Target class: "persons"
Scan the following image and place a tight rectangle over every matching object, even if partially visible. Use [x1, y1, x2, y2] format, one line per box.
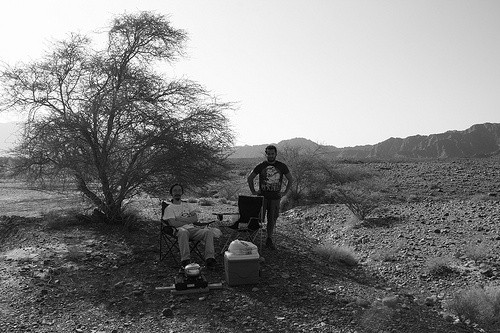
[162, 184, 218, 270]
[249, 145, 293, 247]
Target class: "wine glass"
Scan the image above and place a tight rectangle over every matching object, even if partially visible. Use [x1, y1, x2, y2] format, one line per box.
[217, 214, 223, 226]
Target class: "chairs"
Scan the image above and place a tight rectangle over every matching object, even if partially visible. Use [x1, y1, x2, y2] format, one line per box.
[159, 201, 216, 267]
[218, 195, 264, 256]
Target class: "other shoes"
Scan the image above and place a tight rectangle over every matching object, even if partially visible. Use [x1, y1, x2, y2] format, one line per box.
[267, 238, 278, 249]
[207, 259, 216, 267]
[181, 260, 190, 269]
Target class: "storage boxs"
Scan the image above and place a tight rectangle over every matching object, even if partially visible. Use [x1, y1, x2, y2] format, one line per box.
[222, 250, 261, 279]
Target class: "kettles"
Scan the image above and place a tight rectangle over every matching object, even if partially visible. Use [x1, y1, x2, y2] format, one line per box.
[181, 259, 206, 277]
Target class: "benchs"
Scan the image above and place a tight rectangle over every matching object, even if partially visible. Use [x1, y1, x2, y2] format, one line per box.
[212, 212, 240, 221]
